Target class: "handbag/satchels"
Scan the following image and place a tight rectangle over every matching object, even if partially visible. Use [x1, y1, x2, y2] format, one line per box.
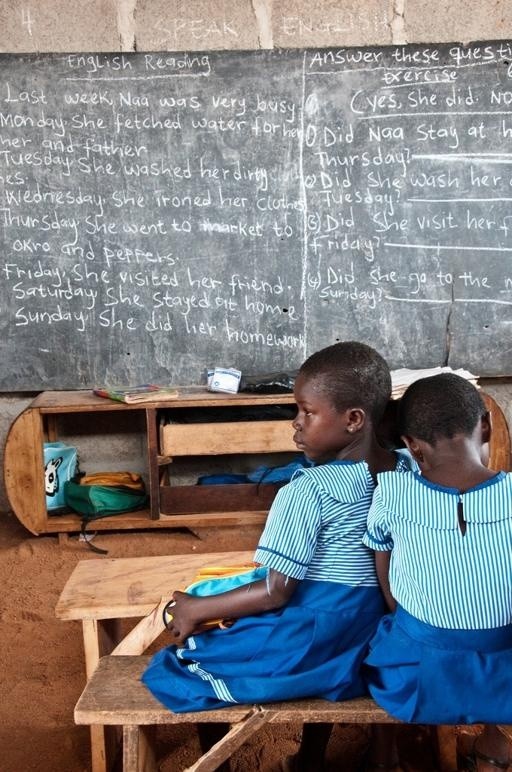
[246, 462, 308, 484]
[43, 442, 79, 511]
[196, 474, 251, 484]
[185, 560, 271, 629]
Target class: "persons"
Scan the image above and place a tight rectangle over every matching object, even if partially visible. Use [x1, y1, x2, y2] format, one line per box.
[142, 342, 412, 713]
[362, 373, 512, 724]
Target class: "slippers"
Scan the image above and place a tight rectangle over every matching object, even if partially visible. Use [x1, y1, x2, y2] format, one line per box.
[363, 756, 402, 772]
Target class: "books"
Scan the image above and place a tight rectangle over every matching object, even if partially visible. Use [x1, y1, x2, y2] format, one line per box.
[84, 380, 178, 404]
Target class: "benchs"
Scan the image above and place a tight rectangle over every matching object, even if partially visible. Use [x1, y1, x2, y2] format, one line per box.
[53, 551, 464, 769]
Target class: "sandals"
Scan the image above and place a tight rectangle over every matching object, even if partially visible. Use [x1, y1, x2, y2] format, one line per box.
[457, 731, 511, 771]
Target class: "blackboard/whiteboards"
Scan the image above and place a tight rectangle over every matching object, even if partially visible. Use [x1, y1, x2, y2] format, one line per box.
[0, 39, 512, 393]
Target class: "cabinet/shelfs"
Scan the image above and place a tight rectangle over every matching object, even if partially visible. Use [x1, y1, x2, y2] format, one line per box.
[5, 380, 511, 545]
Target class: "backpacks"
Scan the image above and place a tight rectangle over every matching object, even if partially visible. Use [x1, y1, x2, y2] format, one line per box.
[64, 471, 149, 519]
